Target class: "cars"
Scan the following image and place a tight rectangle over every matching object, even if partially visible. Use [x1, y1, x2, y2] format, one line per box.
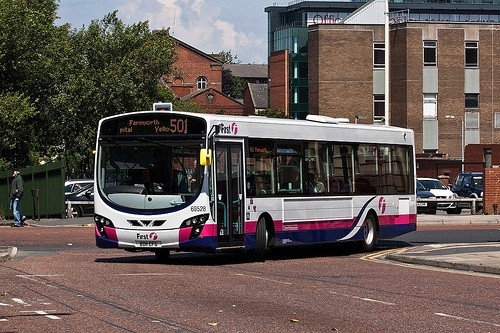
[416, 177, 461, 214]
[65, 178, 94, 195]
[416, 180, 437, 214]
[65, 182, 94, 219]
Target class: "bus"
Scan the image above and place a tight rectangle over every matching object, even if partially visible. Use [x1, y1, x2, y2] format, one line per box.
[93, 102, 417, 263]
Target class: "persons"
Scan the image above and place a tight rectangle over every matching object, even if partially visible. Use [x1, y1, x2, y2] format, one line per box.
[187, 160, 197, 192]
[9, 168, 26, 228]
[308, 173, 325, 193]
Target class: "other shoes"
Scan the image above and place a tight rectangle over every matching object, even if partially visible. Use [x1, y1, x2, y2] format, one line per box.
[11, 225, 21, 227]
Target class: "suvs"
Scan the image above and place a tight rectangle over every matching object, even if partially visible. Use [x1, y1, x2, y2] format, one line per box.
[448, 171, 484, 210]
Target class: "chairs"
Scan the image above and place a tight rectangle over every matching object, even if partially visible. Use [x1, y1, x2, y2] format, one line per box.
[306, 177, 352, 194]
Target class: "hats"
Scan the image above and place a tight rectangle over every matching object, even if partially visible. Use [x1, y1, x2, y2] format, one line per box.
[12, 168, 19, 171]
